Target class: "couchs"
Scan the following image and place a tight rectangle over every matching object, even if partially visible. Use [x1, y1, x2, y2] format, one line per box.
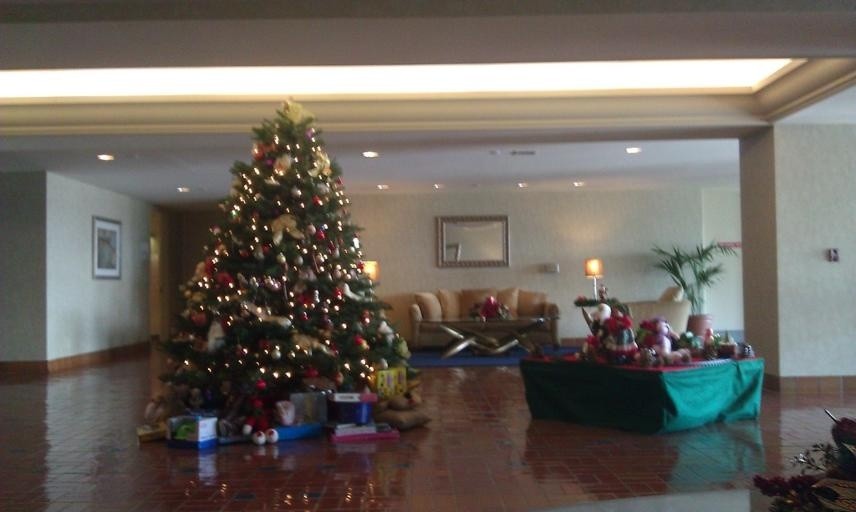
[410, 287, 562, 351]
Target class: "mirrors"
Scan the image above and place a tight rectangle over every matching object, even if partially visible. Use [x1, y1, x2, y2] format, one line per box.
[437, 215, 511, 268]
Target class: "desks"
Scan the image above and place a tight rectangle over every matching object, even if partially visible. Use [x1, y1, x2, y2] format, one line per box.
[519, 356, 766, 435]
[411, 317, 557, 362]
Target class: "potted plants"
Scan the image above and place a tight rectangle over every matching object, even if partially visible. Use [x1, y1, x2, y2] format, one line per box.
[649, 241, 744, 339]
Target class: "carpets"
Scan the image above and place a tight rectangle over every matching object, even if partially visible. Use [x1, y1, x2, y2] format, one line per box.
[405, 345, 580, 370]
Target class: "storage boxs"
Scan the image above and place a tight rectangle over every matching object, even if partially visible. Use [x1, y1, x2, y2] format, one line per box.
[164, 414, 218, 451]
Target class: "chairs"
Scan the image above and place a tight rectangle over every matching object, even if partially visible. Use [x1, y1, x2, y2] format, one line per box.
[624, 285, 692, 334]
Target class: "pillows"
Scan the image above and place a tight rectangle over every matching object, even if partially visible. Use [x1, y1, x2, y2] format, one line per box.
[416, 288, 547, 318]
[656, 286, 684, 302]
[376, 409, 431, 430]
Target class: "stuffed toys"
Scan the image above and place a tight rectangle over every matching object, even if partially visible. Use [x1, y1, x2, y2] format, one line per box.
[580, 304, 756, 369]
[241, 394, 279, 445]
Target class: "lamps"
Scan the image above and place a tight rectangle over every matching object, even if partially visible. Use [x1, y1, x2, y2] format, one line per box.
[363, 259, 376, 282]
[584, 257, 604, 298]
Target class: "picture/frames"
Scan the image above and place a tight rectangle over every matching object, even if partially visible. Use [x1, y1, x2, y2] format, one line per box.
[90, 215, 120, 280]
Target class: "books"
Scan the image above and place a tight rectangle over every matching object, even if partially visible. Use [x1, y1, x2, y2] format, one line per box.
[324, 422, 376, 437]
